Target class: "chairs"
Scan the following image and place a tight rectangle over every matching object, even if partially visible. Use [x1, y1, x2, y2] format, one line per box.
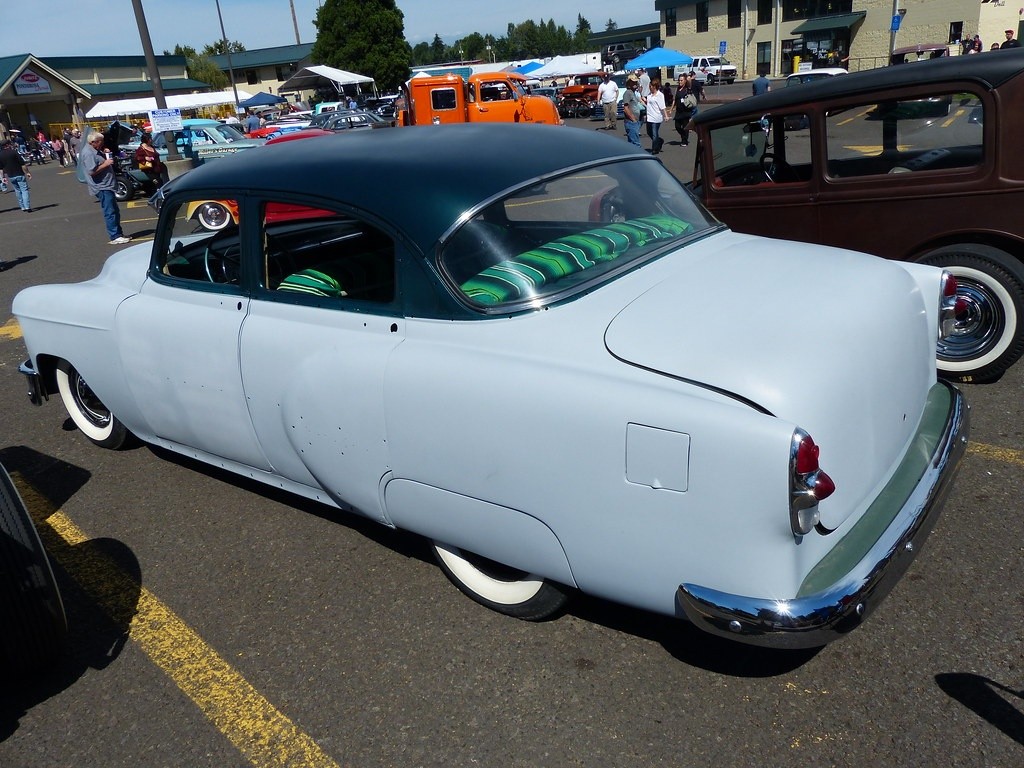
[276, 255, 377, 310]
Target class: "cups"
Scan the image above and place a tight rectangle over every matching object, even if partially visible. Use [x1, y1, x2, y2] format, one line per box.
[105, 152, 113, 159]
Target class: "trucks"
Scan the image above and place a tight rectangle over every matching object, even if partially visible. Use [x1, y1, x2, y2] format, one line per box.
[397, 71, 564, 126]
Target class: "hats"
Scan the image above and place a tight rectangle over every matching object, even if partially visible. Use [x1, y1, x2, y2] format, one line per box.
[625, 74, 639, 83]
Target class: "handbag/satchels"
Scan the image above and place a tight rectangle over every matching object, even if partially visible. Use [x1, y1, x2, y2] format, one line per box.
[139, 161, 152, 169]
[680, 94, 697, 109]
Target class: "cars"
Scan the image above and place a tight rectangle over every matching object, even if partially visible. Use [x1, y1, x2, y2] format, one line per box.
[587, 44, 1024, 385]
[184, 128, 340, 232]
[488, 72, 644, 120]
[9, 119, 971, 652]
[72, 94, 406, 201]
[783, 67, 849, 118]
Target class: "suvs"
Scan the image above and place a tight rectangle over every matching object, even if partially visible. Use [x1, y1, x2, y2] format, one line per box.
[600, 43, 645, 66]
[672, 54, 738, 86]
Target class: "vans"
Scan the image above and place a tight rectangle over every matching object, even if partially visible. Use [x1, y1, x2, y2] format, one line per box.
[886, 43, 954, 117]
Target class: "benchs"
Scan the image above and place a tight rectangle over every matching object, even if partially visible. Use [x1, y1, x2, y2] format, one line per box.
[458, 214, 694, 311]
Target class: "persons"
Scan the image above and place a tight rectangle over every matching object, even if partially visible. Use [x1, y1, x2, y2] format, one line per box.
[11, 127, 82, 168]
[549, 76, 557, 87]
[752, 68, 771, 96]
[827, 50, 850, 67]
[597, 73, 619, 130]
[961, 34, 983, 55]
[226, 111, 240, 124]
[622, 67, 707, 154]
[0, 139, 33, 213]
[82, 132, 133, 244]
[990, 30, 1020, 51]
[134, 133, 163, 197]
[137, 120, 151, 127]
[246, 111, 266, 133]
[566, 74, 576, 86]
[308, 94, 318, 107]
[347, 97, 358, 109]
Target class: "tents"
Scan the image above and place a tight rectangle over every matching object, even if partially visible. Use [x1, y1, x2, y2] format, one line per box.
[398, 71, 430, 94]
[624, 47, 693, 88]
[238, 92, 288, 121]
[277, 66, 379, 102]
[498, 55, 603, 87]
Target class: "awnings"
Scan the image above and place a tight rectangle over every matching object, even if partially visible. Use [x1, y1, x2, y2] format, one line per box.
[790, 13, 866, 35]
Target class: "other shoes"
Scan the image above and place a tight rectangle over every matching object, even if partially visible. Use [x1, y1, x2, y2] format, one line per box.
[26, 209, 32, 212]
[110, 235, 132, 244]
[681, 142, 687, 146]
